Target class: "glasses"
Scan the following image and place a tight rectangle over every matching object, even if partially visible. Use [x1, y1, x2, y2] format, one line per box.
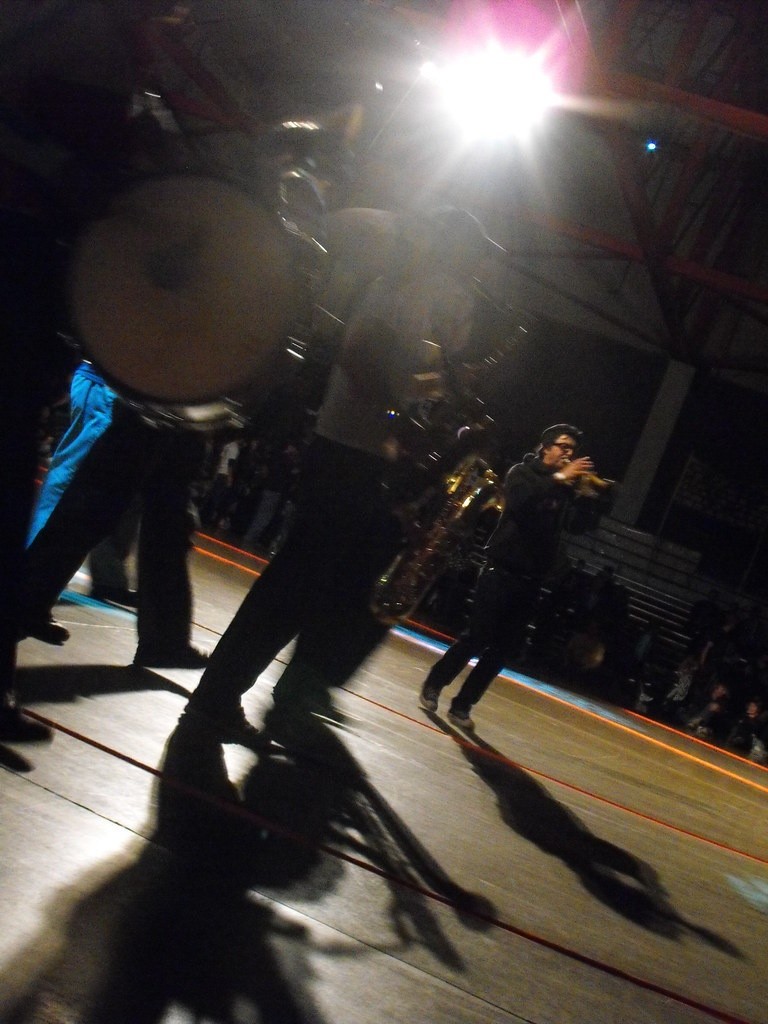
[549, 442, 578, 453]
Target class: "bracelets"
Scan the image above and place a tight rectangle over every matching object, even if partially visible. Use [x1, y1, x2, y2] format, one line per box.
[552, 472, 566, 481]
[421, 338, 441, 368]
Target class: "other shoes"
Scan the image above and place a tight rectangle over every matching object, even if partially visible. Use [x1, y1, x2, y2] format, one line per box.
[90, 586, 138, 607]
[134, 644, 212, 669]
[31, 623, 70, 642]
[272, 672, 345, 722]
[0, 709, 51, 742]
[183, 693, 261, 738]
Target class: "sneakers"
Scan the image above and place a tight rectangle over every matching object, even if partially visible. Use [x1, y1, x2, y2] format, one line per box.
[422, 682, 439, 710]
[447, 707, 474, 729]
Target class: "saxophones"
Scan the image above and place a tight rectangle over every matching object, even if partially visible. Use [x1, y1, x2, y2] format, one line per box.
[366, 450, 507, 623]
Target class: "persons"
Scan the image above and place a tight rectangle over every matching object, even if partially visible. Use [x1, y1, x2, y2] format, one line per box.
[0, 0, 503, 754]
[421, 421, 597, 732]
[506, 555, 768, 767]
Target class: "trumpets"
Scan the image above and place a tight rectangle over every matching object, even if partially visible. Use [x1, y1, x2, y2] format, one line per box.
[558, 457, 616, 496]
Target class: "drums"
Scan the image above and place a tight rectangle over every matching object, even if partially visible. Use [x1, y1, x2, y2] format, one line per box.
[69, 175, 297, 399]
